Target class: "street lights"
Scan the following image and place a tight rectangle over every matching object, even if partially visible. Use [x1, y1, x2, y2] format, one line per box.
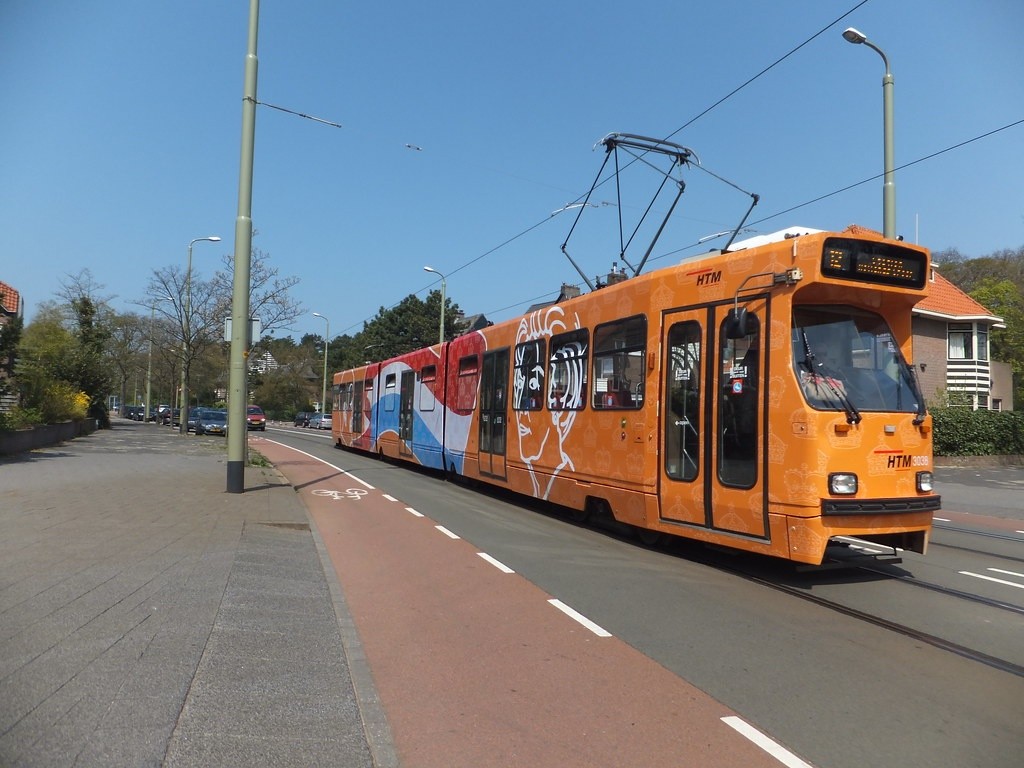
[312, 313, 330, 414]
[146, 298, 175, 422]
[424, 266, 449, 346]
[842, 27, 897, 241]
[225, 97, 343, 493]
[178, 237, 222, 434]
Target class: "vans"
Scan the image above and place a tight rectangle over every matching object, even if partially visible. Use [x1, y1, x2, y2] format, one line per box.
[246, 405, 266, 432]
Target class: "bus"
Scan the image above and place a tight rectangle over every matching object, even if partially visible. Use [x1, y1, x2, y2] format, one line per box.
[332, 132, 943, 566]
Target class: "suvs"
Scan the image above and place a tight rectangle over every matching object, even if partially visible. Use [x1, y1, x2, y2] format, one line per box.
[294, 412, 316, 429]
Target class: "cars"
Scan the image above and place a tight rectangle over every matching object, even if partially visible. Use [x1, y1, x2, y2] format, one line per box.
[123, 405, 180, 426]
[186, 407, 209, 432]
[195, 411, 227, 436]
[308, 414, 333, 431]
